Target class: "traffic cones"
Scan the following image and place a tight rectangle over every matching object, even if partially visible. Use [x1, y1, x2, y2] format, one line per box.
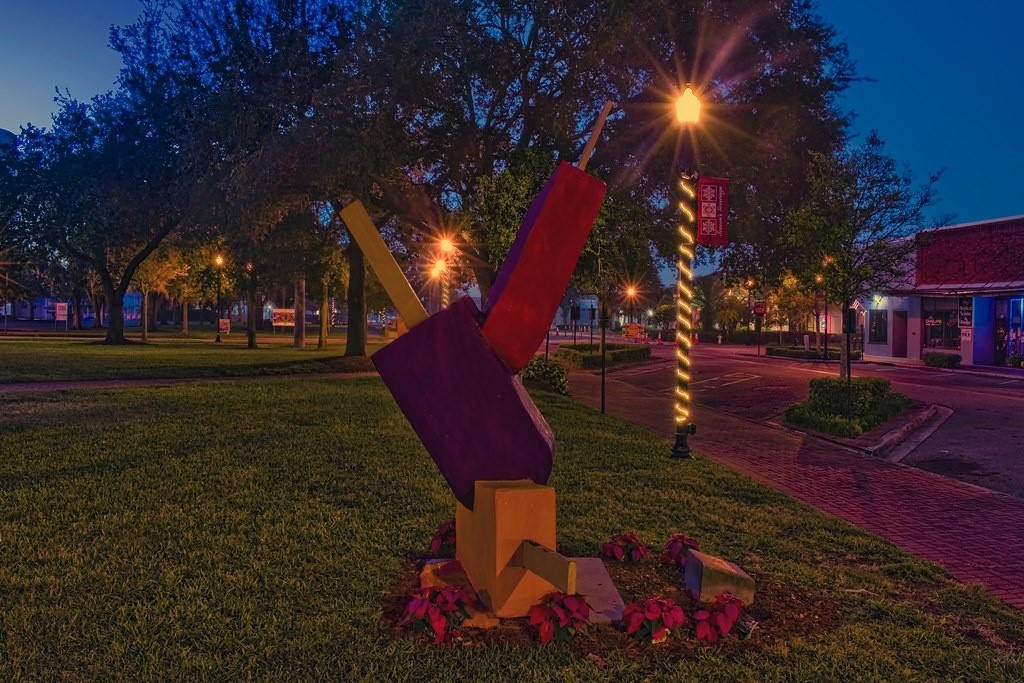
[555, 331, 559, 336]
[656, 334, 664, 345]
[673, 335, 681, 347]
[693, 334, 699, 345]
[642, 333, 649, 344]
[635, 332, 641, 343]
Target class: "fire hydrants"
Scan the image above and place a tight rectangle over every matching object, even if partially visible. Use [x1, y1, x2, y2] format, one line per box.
[716, 335, 722, 344]
[793, 338, 799, 349]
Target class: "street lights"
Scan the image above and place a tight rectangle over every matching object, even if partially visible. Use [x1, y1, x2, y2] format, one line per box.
[214, 256, 223, 343]
[628, 287, 634, 323]
[746, 280, 754, 331]
[668, 86, 704, 462]
[431, 239, 455, 312]
